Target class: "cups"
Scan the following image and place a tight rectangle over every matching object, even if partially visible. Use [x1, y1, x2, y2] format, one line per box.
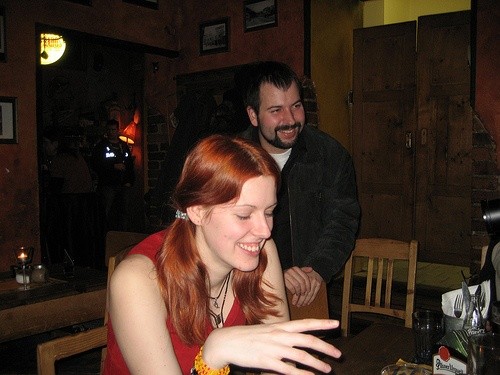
[412, 311, 445, 366]
[379, 363, 426, 375]
[466, 331, 500, 375]
[441, 314, 465, 336]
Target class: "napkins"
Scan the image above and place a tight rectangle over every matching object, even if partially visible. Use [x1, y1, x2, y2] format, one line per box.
[440, 279, 491, 320]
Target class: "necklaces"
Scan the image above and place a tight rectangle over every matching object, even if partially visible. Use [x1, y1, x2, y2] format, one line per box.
[207, 271, 231, 328]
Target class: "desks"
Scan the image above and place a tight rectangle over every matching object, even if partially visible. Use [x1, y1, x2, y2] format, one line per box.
[311, 322, 417, 375]
[0, 261, 107, 343]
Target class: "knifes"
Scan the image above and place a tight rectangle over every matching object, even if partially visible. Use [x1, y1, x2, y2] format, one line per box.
[464, 284, 481, 326]
[461, 280, 472, 313]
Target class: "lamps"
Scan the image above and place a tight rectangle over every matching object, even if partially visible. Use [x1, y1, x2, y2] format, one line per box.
[119, 121, 136, 146]
[39, 32, 67, 65]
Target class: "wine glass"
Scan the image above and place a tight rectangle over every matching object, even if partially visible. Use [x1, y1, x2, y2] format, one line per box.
[13, 234, 37, 290]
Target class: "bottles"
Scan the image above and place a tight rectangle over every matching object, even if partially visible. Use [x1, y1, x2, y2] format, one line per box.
[30, 265, 49, 284]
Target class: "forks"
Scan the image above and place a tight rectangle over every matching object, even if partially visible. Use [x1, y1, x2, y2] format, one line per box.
[454, 294, 463, 318]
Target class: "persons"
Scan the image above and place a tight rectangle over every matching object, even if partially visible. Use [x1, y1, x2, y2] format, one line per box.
[98, 119, 136, 232]
[104, 136, 341, 375]
[239, 61, 361, 308]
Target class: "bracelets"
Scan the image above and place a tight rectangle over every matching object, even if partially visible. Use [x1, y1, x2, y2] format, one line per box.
[190, 345, 230, 375]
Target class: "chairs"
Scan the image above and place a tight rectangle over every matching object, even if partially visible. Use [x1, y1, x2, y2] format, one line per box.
[340, 238, 418, 337]
[36, 231, 150, 375]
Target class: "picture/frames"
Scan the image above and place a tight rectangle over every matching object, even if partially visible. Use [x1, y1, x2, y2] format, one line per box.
[0, 96, 19, 144]
[242, 0, 278, 33]
[0, 6, 8, 63]
[199, 16, 228, 55]
